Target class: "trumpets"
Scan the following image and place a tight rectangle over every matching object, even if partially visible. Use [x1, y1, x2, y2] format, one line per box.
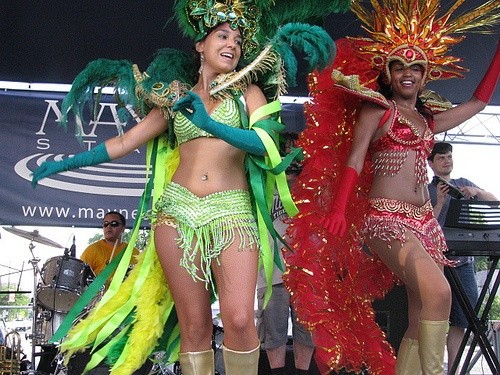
[0, 330, 21, 374]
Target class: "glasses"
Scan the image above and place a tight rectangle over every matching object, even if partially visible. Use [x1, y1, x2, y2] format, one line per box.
[101, 220, 123, 228]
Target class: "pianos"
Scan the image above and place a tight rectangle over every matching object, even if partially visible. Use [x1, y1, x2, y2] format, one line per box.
[436, 225, 500, 375]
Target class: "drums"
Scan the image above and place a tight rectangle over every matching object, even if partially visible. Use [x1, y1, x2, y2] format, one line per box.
[33, 304, 87, 348]
[62, 311, 163, 375]
[37, 255, 99, 313]
[124, 265, 133, 283]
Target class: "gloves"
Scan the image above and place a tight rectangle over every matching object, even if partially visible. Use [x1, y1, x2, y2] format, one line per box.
[31, 140, 112, 187]
[172, 90, 269, 156]
[473, 43, 500, 103]
[322, 166, 359, 236]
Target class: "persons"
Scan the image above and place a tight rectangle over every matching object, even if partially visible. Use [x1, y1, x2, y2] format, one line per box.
[427, 142, 500, 375]
[80, 211, 140, 286]
[257, 131, 317, 375]
[30, 0, 337, 375]
[281, 0, 500, 375]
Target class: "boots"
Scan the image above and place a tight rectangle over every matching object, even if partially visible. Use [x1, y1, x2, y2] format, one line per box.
[222, 338, 262, 375]
[396, 336, 424, 375]
[418, 319, 448, 375]
[178, 349, 214, 375]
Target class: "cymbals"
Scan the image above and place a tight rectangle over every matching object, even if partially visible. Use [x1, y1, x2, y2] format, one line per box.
[1, 225, 66, 250]
